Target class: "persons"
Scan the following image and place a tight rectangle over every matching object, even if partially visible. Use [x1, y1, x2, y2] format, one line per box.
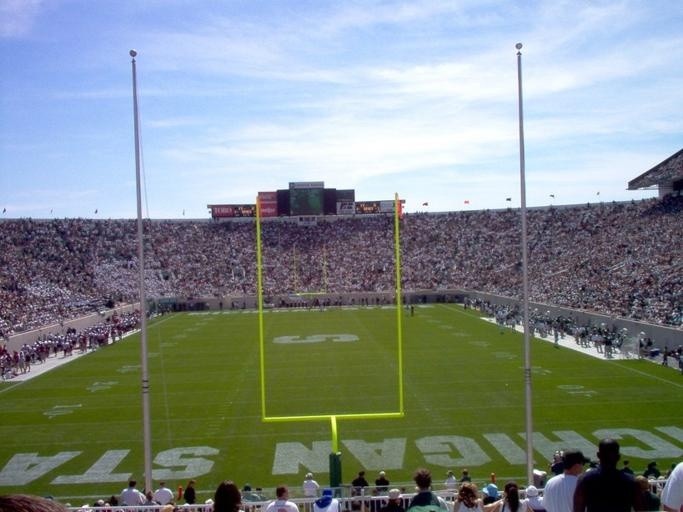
[470, 192, 683, 372]
[213, 439, 683, 512]
[0, 216, 174, 380]
[238, 206, 243, 216]
[174, 214, 470, 316]
[1, 480, 214, 512]
[251, 206, 255, 215]
[372, 203, 380, 214]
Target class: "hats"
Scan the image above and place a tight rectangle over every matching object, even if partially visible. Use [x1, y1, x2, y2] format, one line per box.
[525, 485, 538, 497]
[562, 452, 590, 469]
[388, 489, 402, 499]
[482, 483, 500, 498]
[305, 472, 313, 478]
[322, 488, 333, 495]
